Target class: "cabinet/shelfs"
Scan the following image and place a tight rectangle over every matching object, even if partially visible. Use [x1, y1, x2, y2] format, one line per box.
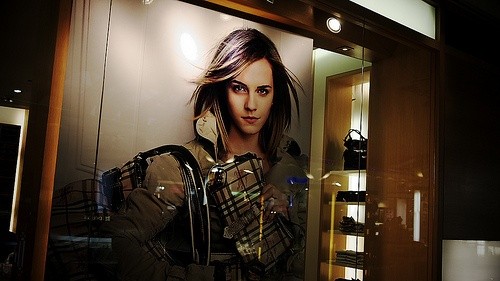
[328, 170, 366, 281]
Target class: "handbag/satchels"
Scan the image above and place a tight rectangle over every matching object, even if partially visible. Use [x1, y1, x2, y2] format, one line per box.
[204, 150, 301, 279]
[342, 128, 367, 152]
[47, 144, 209, 281]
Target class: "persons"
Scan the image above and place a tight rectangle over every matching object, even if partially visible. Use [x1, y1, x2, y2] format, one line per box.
[102, 26, 310, 281]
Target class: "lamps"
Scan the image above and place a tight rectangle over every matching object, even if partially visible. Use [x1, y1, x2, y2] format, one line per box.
[326, 17, 345, 34]
